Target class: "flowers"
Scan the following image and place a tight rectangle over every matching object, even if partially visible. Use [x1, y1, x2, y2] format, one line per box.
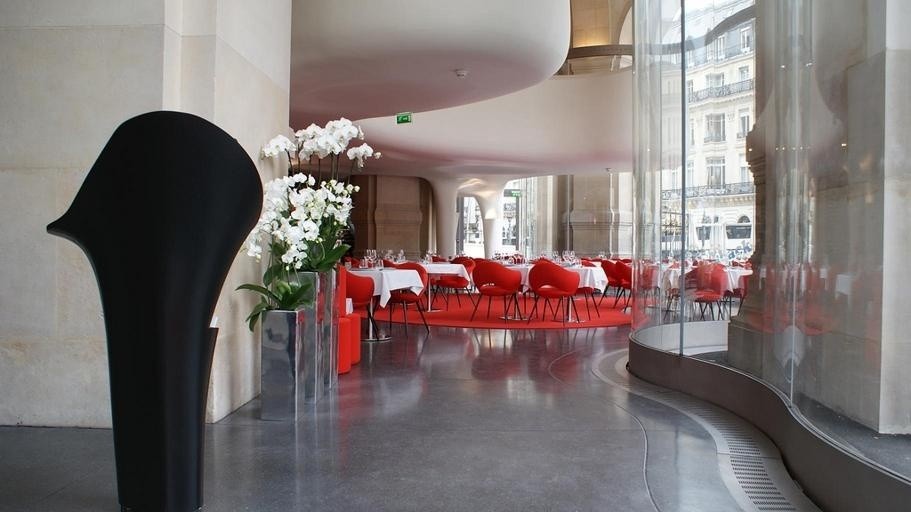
[234, 117, 382, 332]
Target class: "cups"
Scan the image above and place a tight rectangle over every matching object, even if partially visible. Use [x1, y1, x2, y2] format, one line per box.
[344, 261, 353, 271]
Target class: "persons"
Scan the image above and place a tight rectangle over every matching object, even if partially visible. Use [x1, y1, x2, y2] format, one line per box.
[342, 217, 356, 267]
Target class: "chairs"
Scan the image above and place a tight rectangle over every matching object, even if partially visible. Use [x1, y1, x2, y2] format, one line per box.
[297, 253, 758, 374]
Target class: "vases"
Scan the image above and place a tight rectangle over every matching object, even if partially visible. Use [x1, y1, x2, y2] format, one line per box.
[258, 264, 341, 422]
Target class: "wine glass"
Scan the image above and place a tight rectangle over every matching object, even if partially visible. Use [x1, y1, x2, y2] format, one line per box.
[359, 248, 433, 271]
[491, 249, 583, 268]
